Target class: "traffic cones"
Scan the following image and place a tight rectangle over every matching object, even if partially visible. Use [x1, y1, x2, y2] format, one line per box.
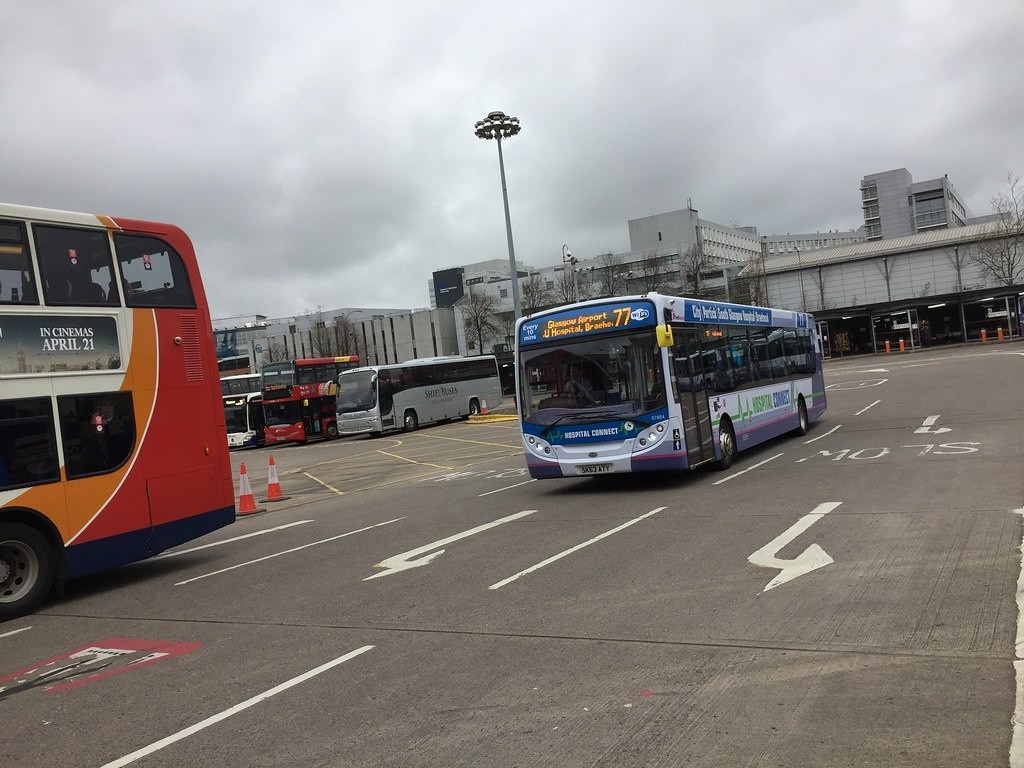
[234, 462, 267, 517]
[259, 455, 291, 503]
[481, 400, 488, 415]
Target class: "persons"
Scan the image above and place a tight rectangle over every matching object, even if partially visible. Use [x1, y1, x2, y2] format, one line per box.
[563, 366, 595, 407]
[784, 360, 797, 375]
[944, 323, 949, 343]
[920, 319, 930, 347]
[21, 247, 131, 304]
[41, 427, 75, 479]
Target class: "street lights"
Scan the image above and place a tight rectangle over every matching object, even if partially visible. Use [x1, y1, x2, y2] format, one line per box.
[346, 310, 362, 327]
[475, 112, 522, 414]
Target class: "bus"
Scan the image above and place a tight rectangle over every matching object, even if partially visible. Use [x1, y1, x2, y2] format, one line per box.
[219, 371, 293, 447]
[335, 352, 502, 437]
[0, 201, 236, 622]
[260, 356, 359, 445]
[515, 293, 828, 479]
